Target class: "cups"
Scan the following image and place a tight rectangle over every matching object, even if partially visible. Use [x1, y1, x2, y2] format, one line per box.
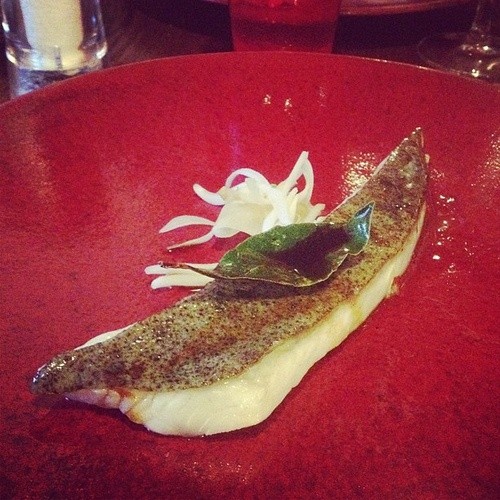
[0, 0, 107, 72]
[229, 0, 338, 54]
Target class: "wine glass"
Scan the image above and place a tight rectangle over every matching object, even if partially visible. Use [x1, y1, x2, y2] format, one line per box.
[418, 0, 499, 82]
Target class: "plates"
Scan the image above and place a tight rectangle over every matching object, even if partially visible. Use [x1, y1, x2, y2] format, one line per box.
[1, 52, 499, 500]
